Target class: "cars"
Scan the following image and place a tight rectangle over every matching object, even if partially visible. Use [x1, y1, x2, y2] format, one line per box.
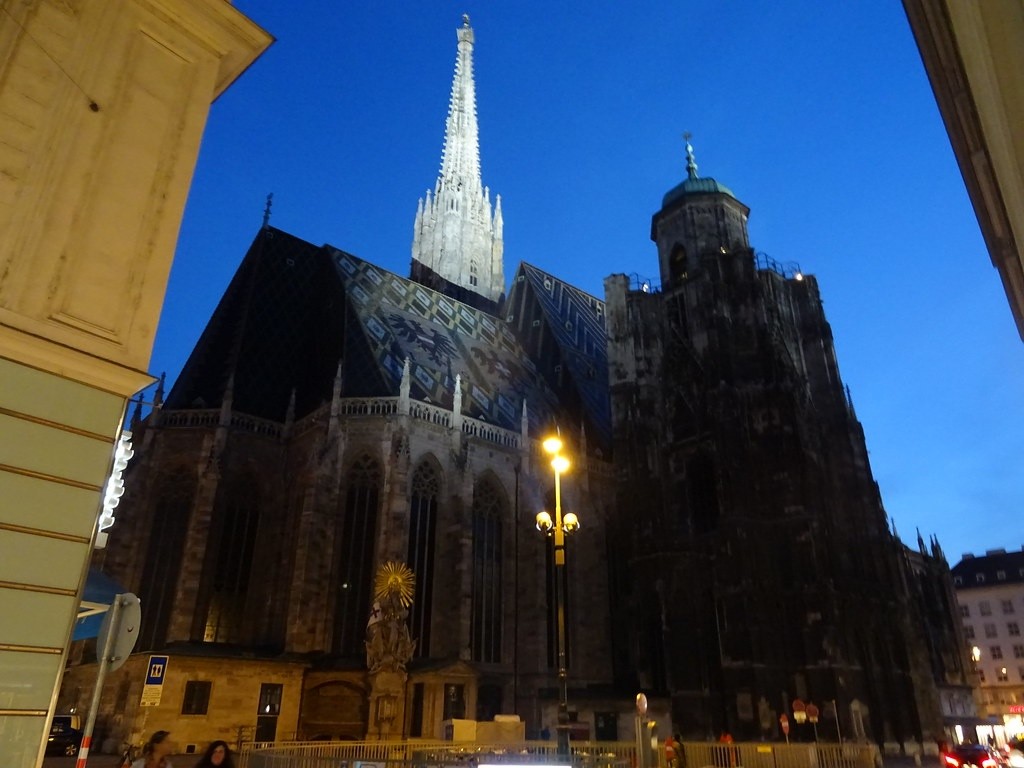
[944, 743, 1007, 768]
[45, 725, 83, 758]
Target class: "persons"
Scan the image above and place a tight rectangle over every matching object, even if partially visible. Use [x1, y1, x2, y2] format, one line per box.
[665, 734, 685, 768]
[196, 740, 235, 768]
[368, 577, 410, 669]
[707, 730, 716, 742]
[719, 730, 733, 744]
[130, 730, 173, 768]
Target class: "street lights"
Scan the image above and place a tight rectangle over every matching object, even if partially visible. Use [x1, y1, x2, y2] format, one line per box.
[533, 429, 582, 765]
